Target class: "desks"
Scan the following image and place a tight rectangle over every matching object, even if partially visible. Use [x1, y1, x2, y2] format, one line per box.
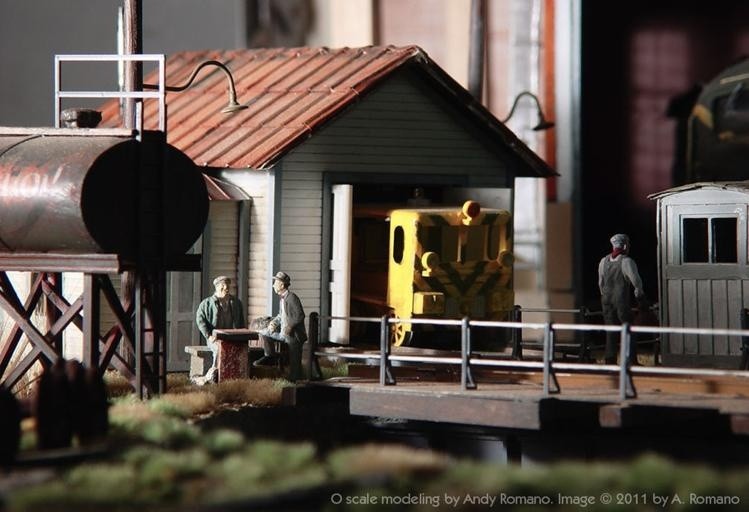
[210, 328, 259, 385]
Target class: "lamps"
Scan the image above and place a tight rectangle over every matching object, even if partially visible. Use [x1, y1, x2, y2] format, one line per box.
[500, 89, 556, 133]
[139, 59, 250, 117]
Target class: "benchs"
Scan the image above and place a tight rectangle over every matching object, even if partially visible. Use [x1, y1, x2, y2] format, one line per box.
[183, 345, 265, 382]
